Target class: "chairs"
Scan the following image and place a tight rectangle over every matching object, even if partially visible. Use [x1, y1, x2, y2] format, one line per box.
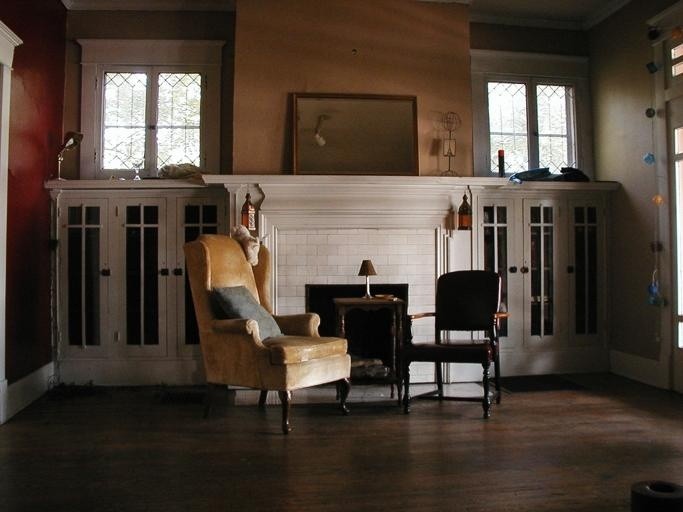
[401, 270, 503, 420]
[183, 231, 353, 435]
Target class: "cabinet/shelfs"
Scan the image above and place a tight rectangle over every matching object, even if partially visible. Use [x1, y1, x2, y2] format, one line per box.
[477, 198, 560, 351]
[56, 197, 109, 360]
[561, 198, 605, 351]
[109, 195, 229, 360]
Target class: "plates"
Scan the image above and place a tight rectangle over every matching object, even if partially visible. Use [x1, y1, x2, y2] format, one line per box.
[375, 294, 393, 299]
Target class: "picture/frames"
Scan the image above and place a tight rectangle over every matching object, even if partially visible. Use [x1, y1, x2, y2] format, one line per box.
[288, 91, 421, 174]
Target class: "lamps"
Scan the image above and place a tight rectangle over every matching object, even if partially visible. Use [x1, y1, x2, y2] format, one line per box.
[357, 259, 378, 300]
[54, 129, 82, 178]
[239, 193, 259, 233]
[442, 109, 463, 177]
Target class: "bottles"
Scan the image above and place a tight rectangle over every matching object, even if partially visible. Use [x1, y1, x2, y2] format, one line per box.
[241, 192, 259, 236]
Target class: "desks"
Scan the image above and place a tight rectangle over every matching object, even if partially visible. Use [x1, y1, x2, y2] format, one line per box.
[333, 296, 407, 414]
